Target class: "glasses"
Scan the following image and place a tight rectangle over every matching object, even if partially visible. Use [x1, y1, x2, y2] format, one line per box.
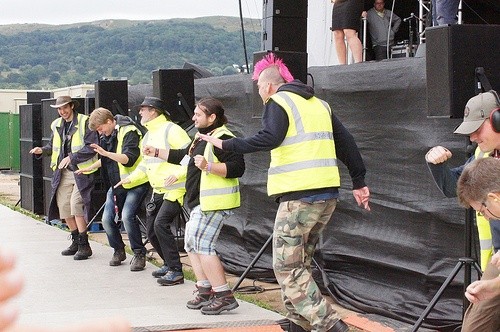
[477, 192, 493, 216]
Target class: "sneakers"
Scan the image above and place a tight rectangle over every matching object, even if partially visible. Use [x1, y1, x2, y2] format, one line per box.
[157, 270, 184, 286]
[152, 265, 170, 278]
[110, 247, 126, 265]
[130, 253, 146, 271]
[200, 289, 239, 315]
[186, 284, 214, 310]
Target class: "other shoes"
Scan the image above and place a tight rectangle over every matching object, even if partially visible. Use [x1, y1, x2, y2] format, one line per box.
[326, 319, 348, 332]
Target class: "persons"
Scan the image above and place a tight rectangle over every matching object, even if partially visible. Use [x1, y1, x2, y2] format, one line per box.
[457, 157, 500, 304]
[0, 249, 26, 332]
[425, 89, 500, 332]
[330, 0, 368, 64]
[141, 98, 246, 315]
[113, 96, 193, 285]
[431, 0, 460, 27]
[29, 97, 99, 261]
[75, 107, 151, 271]
[198, 52, 372, 332]
[362, 0, 402, 61]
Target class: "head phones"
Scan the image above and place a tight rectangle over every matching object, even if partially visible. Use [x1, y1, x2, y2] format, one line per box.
[489, 90, 500, 133]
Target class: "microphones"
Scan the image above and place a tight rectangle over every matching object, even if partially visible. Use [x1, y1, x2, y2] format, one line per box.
[467, 141, 477, 151]
[403, 16, 413, 22]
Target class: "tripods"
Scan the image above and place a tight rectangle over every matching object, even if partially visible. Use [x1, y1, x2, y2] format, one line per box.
[407, 118, 484, 332]
[231, 228, 323, 293]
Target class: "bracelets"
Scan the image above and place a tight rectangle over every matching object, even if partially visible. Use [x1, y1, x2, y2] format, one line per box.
[205, 162, 211, 173]
[154, 148, 159, 157]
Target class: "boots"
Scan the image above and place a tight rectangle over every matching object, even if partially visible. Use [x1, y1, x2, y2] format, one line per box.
[61, 230, 80, 256]
[75, 234, 92, 260]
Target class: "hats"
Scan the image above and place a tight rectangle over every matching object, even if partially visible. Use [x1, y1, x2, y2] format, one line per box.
[50, 95, 80, 109]
[133, 96, 170, 116]
[454, 92, 498, 134]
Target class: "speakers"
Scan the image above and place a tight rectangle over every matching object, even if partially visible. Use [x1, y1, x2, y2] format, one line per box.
[426, 23, 499, 119]
[152, 68, 195, 128]
[94, 79, 128, 116]
[18, 97, 106, 218]
[251, 0, 308, 119]
